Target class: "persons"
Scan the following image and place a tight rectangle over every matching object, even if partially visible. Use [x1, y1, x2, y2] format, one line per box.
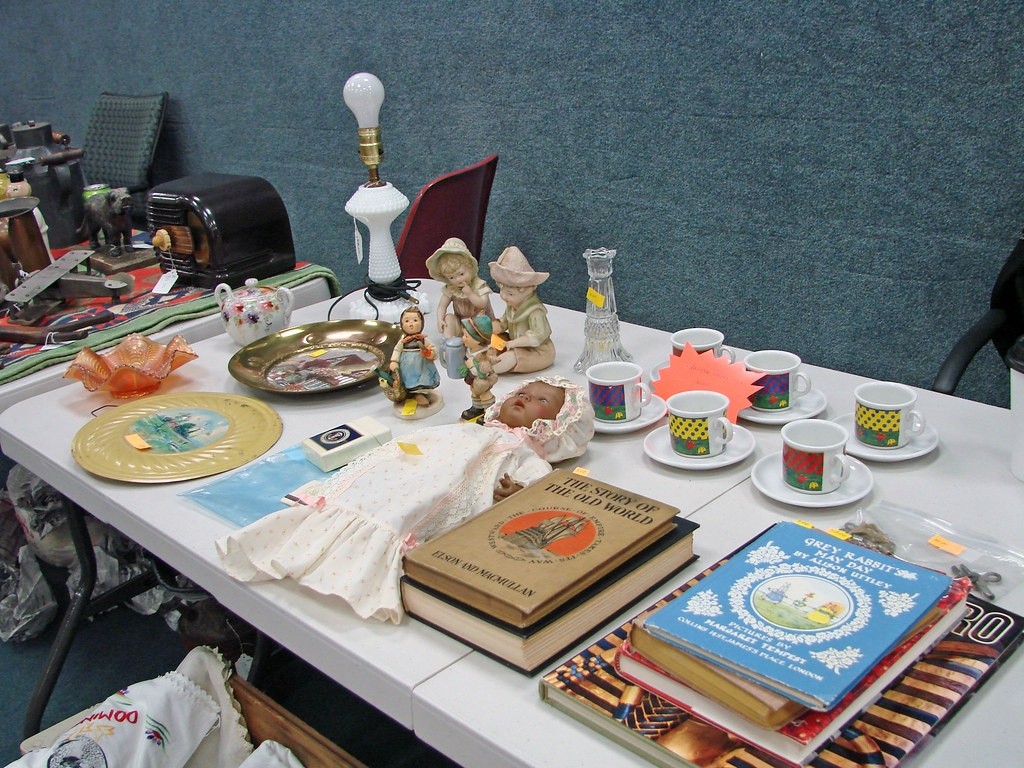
[214, 376, 596, 624]
[488, 246, 556, 373]
[426, 238, 496, 338]
[390, 308, 440, 399]
[460, 315, 499, 425]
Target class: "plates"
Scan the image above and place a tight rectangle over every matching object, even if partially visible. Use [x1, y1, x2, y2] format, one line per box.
[227, 318, 406, 396]
[642, 422, 757, 470]
[650, 360, 669, 382]
[830, 411, 940, 462]
[750, 450, 874, 508]
[737, 387, 828, 425]
[592, 392, 667, 434]
[62, 332, 199, 400]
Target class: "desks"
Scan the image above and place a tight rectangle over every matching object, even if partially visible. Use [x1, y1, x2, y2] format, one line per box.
[0, 237, 332, 614]
[413, 382, 1024, 768]
[0, 271, 879, 747]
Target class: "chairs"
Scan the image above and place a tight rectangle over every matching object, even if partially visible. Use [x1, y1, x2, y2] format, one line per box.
[73, 89, 175, 240]
[394, 153, 502, 281]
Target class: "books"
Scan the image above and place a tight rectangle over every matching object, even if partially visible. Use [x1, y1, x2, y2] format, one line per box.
[399, 467, 1024, 768]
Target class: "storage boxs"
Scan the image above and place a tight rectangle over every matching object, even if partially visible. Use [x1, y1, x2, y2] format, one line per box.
[298, 417, 392, 472]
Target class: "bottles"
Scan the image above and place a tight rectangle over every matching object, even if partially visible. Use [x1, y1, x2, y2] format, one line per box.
[5, 170, 32, 199]
[572, 247, 635, 374]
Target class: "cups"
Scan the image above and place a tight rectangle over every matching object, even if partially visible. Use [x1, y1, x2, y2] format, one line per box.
[780, 419, 851, 495]
[665, 390, 734, 459]
[670, 327, 736, 366]
[585, 361, 652, 424]
[853, 380, 926, 450]
[743, 350, 811, 413]
